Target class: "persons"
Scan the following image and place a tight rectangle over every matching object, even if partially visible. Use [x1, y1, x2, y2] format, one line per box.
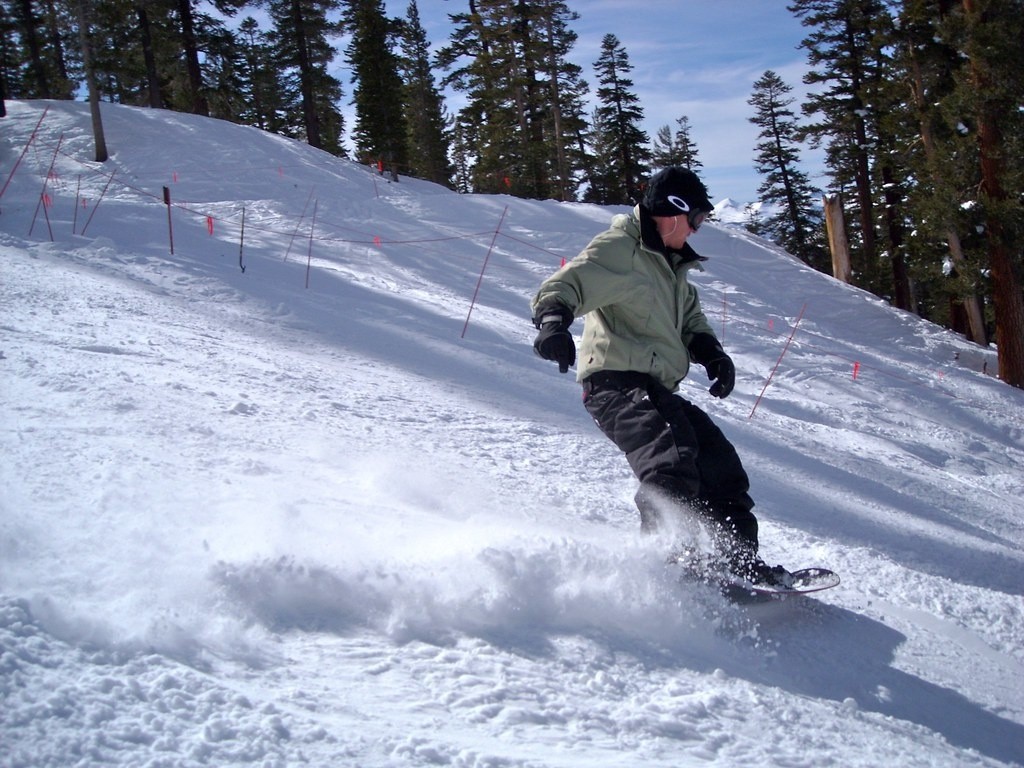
[532, 167, 796, 588]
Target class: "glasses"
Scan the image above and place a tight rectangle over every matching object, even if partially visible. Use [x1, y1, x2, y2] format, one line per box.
[683, 208, 710, 230]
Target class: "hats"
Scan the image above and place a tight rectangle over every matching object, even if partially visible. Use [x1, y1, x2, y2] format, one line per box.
[643, 166, 715, 218]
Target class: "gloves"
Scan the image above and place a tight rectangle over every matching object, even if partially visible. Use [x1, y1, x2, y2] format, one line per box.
[693, 333, 735, 400]
[533, 313, 576, 373]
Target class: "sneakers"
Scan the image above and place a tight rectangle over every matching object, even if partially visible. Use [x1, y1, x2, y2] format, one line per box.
[725, 551, 783, 586]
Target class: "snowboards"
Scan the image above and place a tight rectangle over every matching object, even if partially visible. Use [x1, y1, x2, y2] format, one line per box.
[698, 557, 841, 594]
